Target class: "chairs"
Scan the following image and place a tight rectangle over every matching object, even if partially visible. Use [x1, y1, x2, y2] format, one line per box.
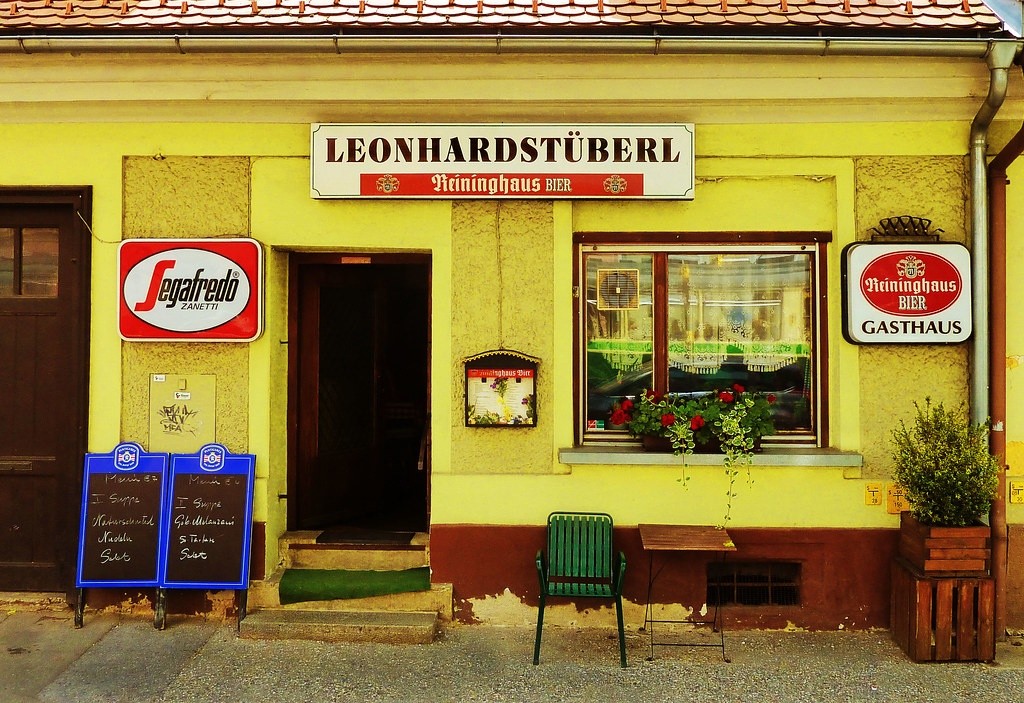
[533, 511, 628, 669]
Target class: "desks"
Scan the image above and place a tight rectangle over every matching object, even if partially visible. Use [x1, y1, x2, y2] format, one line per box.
[637, 523, 738, 664]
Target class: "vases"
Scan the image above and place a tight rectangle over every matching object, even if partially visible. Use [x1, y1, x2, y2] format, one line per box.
[639, 429, 764, 453]
[898, 512, 993, 577]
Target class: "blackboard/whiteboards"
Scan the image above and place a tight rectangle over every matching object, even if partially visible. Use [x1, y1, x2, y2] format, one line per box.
[75, 451, 257, 590]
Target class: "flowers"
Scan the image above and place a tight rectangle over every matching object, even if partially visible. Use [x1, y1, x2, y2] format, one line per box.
[888, 394, 1006, 526]
[611, 383, 778, 531]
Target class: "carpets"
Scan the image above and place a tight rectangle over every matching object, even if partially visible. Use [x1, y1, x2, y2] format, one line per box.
[278, 567, 432, 605]
[318, 527, 416, 545]
